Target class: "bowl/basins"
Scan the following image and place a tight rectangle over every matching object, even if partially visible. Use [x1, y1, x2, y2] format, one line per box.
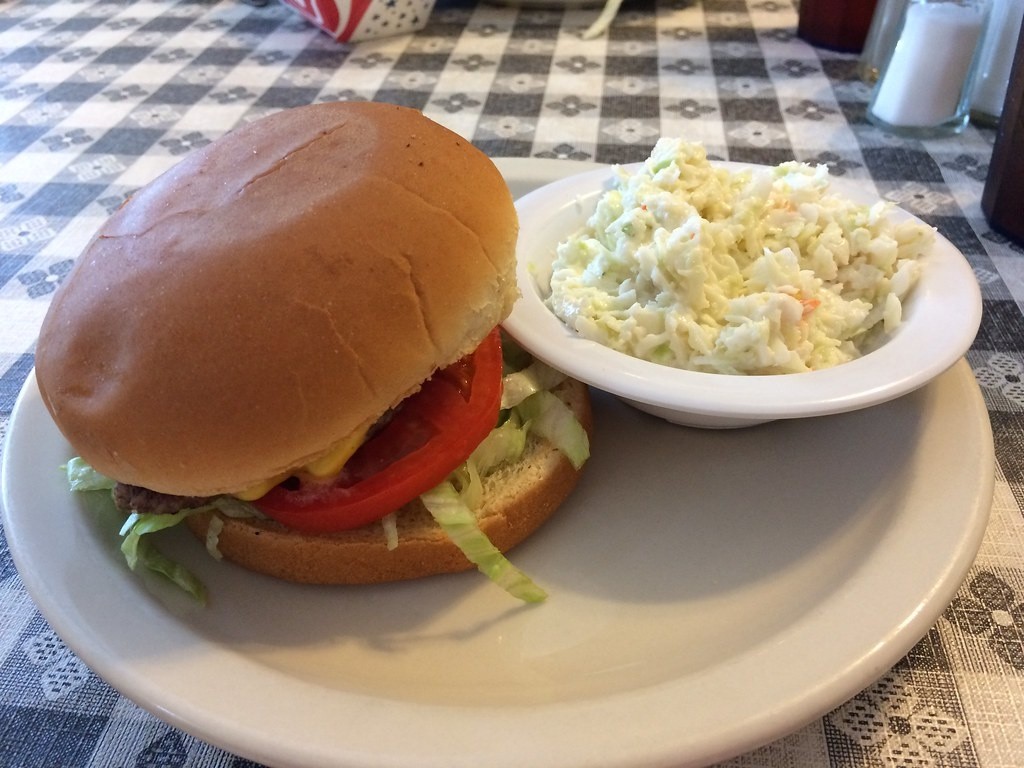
[498, 162, 984, 429]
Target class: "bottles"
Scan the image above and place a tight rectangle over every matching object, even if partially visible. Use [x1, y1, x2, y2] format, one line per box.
[866, 0, 987, 138]
[970, 0, 1024, 127]
[857, 0, 909, 89]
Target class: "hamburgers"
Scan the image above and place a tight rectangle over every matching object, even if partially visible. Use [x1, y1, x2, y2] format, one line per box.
[32, 104, 591, 611]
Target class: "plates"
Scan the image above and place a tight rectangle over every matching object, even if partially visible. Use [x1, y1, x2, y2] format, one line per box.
[2, 355, 996, 767]
